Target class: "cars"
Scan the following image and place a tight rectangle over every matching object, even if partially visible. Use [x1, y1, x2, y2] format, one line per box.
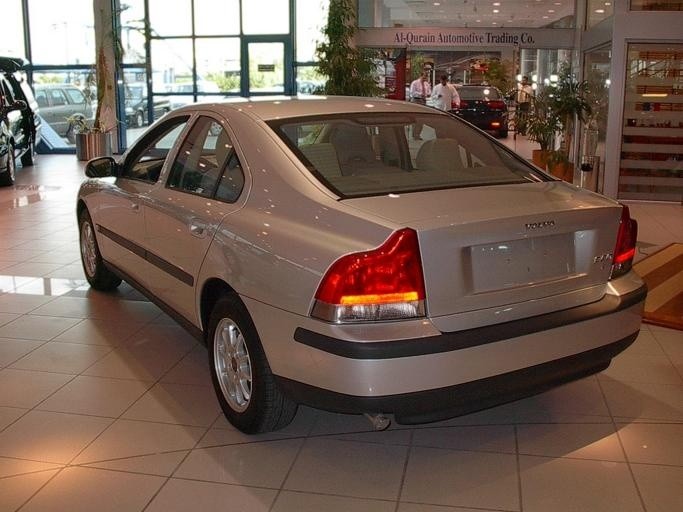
[77, 95, 644, 437]
[449, 82, 509, 138]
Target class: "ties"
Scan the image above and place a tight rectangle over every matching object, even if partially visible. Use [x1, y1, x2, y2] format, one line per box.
[421, 81, 425, 101]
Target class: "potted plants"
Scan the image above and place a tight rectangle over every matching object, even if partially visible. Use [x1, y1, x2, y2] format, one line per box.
[504, 62, 596, 182]
[66, 113, 129, 161]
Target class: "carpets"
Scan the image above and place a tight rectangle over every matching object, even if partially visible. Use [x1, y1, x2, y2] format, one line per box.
[631, 242, 682, 330]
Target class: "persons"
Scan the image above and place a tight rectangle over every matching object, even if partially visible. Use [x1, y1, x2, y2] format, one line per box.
[410, 71, 432, 142]
[518, 76, 532, 136]
[405, 77, 488, 102]
[430, 75, 461, 138]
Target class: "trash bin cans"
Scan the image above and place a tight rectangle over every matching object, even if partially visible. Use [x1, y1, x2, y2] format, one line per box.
[581, 155, 599, 192]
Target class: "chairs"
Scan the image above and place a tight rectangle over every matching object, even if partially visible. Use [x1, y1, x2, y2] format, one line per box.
[197, 118, 466, 209]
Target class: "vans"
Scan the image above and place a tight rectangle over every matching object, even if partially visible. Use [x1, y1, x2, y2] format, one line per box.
[34, 77, 322, 146]
[0, 56, 42, 187]
